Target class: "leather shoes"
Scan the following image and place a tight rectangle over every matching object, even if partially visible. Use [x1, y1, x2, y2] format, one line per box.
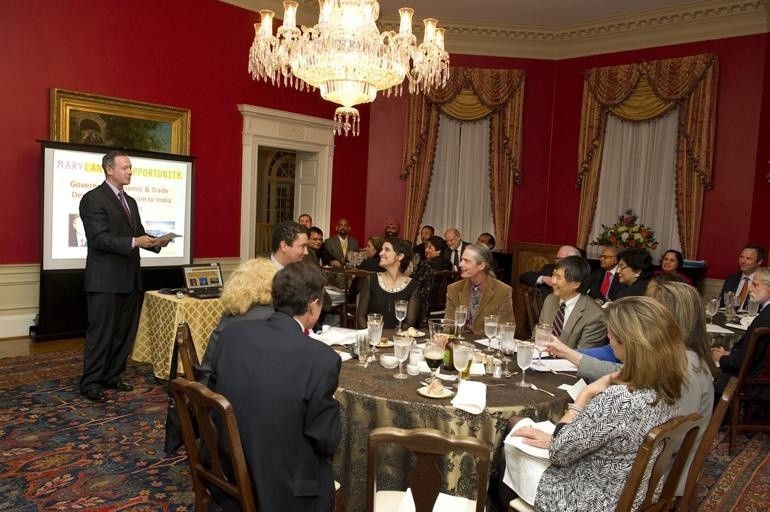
[81, 389, 106, 401]
[106, 379, 132, 391]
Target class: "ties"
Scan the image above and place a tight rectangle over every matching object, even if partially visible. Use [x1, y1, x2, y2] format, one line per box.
[552, 303, 565, 338]
[118, 190, 133, 225]
[470, 287, 479, 320]
[341, 240, 347, 260]
[600, 272, 610, 298]
[454, 249, 459, 269]
[80, 239, 84, 246]
[736, 277, 749, 304]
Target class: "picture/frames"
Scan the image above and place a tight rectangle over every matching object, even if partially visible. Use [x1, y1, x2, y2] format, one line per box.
[511, 241, 561, 341]
[50, 88, 191, 156]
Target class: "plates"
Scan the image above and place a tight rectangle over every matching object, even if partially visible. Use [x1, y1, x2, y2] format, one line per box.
[401, 328, 426, 339]
[417, 383, 453, 399]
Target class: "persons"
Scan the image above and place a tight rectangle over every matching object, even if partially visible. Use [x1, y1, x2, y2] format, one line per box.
[519, 244, 691, 352]
[298, 214, 496, 313]
[202, 220, 343, 512]
[70, 215, 88, 246]
[357, 238, 421, 329]
[78, 148, 175, 403]
[442, 242, 515, 333]
[504, 282, 721, 512]
[707, 244, 770, 403]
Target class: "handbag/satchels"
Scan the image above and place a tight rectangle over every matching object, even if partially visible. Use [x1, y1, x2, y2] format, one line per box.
[165, 403, 200, 452]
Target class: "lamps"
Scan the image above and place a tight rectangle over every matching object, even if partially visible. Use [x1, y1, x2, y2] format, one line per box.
[248, 0, 450, 137]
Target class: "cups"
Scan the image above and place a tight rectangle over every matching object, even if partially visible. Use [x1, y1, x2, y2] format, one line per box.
[428, 317, 457, 349]
[454, 304, 467, 342]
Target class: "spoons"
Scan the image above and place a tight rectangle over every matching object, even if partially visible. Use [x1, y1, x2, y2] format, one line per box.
[529, 381, 556, 399]
[550, 366, 578, 379]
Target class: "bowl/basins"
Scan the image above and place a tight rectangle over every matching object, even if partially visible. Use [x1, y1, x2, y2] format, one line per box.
[378, 352, 399, 371]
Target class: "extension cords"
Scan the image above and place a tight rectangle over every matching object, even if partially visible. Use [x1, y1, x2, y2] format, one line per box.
[177, 291, 184, 299]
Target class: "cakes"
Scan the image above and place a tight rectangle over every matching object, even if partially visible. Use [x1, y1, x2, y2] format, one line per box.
[407, 325, 419, 337]
[427, 379, 445, 396]
[379, 336, 389, 347]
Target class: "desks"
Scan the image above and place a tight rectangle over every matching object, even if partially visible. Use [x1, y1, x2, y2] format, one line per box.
[131, 290, 224, 384]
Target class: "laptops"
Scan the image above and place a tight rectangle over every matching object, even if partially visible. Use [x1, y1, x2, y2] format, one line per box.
[181, 263, 224, 299]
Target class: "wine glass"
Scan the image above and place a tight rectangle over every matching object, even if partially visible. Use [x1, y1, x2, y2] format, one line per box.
[423, 342, 445, 387]
[482, 315, 516, 377]
[514, 341, 533, 388]
[452, 339, 471, 389]
[393, 335, 410, 380]
[394, 300, 408, 338]
[348, 250, 367, 272]
[355, 334, 371, 366]
[413, 253, 420, 274]
[367, 313, 385, 362]
[533, 323, 554, 368]
[705, 290, 759, 329]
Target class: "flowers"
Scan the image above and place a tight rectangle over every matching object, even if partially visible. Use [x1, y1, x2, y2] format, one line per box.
[589, 208, 659, 251]
